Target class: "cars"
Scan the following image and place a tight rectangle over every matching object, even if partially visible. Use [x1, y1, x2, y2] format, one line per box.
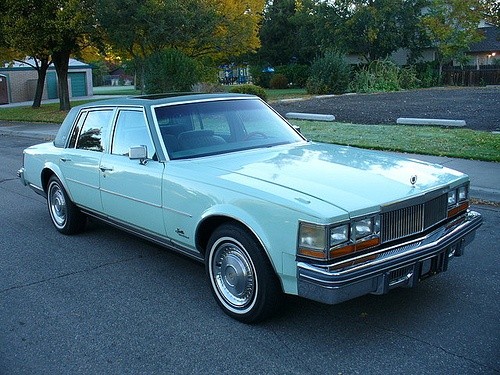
[16, 91, 482, 324]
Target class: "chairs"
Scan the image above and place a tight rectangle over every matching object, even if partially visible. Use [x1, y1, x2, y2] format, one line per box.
[157, 128, 226, 152]
